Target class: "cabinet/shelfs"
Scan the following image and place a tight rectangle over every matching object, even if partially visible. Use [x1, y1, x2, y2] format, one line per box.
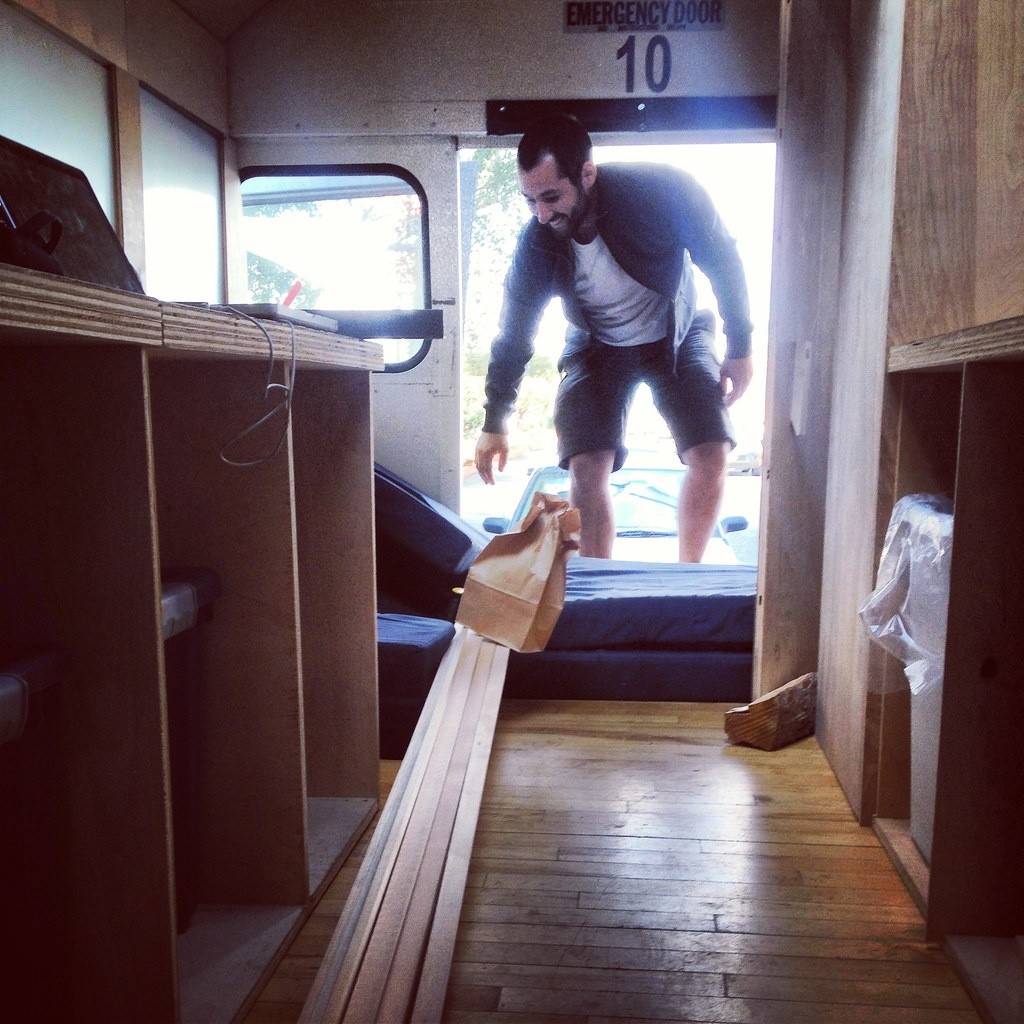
[0, 254, 382, 1024]
[872, 311, 1024, 1024]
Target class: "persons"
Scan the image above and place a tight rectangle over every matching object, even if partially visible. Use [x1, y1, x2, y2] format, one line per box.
[475, 115, 753, 561]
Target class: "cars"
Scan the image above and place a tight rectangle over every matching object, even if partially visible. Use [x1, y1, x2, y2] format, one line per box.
[491, 462, 749, 565]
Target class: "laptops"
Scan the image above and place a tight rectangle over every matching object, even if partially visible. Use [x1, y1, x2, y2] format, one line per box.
[0, 136, 340, 331]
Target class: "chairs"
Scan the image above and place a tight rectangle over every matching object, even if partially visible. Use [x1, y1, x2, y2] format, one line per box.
[373, 458, 757, 703]
